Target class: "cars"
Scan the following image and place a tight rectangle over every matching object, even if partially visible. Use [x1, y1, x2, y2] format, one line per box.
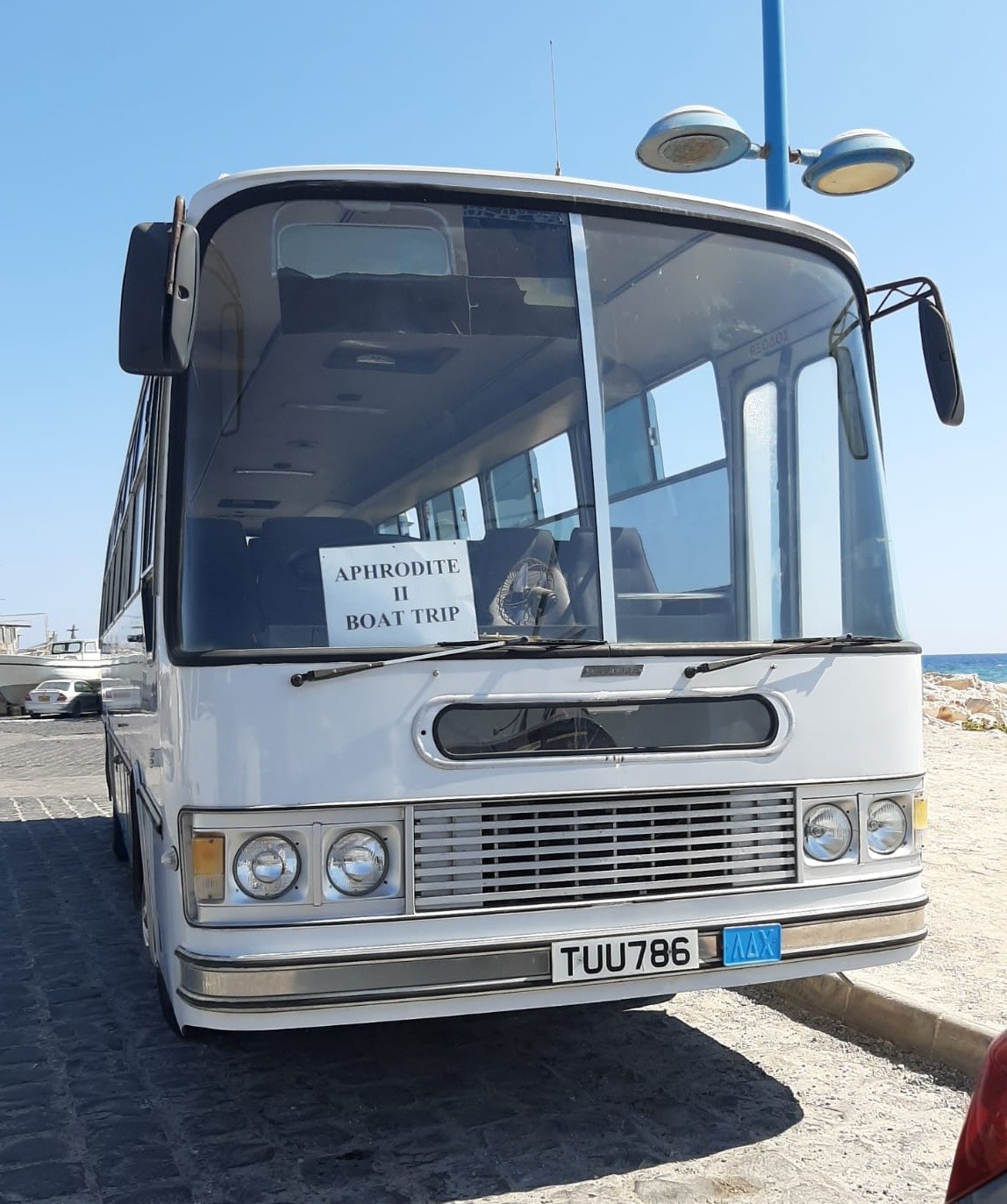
[24, 679, 101, 719]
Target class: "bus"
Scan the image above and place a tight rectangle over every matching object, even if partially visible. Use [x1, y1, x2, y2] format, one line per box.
[98, 163, 968, 1036]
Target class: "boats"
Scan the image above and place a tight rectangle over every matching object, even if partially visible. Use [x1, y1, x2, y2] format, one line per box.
[0, 597, 98, 718]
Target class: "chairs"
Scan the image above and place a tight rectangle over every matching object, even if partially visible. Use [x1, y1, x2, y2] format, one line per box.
[188, 514, 658, 642]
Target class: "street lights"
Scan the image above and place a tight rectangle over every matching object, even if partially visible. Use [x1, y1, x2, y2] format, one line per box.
[636, 1, 918, 640]
